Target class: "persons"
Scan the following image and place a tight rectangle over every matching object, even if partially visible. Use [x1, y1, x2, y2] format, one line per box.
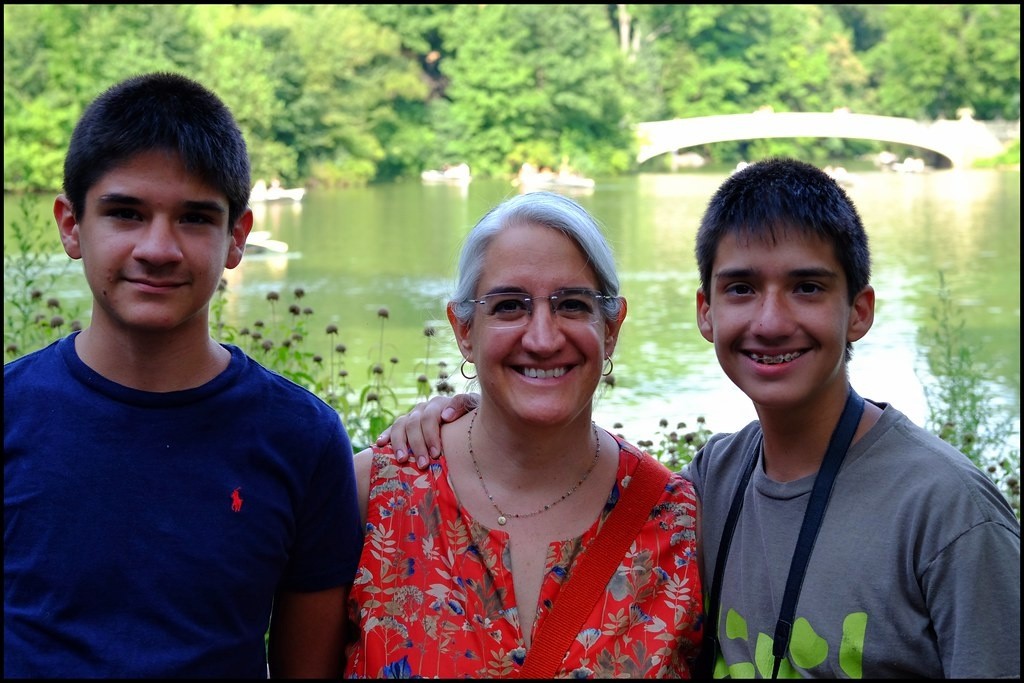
[376, 159, 1020, 679]
[342, 191, 706, 679]
[4, 73, 363, 681]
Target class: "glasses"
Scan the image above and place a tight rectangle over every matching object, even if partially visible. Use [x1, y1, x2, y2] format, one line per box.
[467, 289, 617, 329]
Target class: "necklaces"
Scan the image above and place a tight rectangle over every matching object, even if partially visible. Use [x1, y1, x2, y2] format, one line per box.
[467, 408, 600, 526]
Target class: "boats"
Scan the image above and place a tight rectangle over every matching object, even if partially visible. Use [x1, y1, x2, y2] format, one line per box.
[514, 161, 596, 191]
[248, 178, 306, 201]
[244, 231, 289, 258]
[421, 162, 473, 183]
